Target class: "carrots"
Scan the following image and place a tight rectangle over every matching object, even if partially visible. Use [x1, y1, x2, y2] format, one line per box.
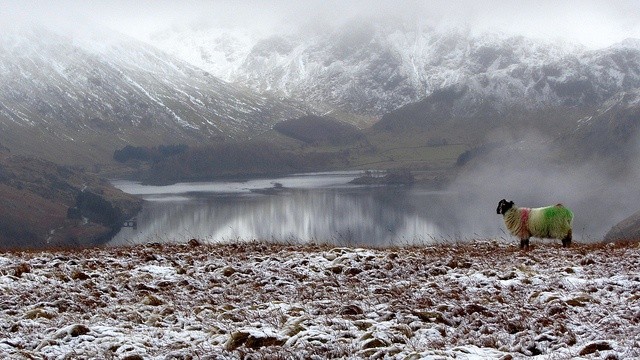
[497, 199, 574, 250]
